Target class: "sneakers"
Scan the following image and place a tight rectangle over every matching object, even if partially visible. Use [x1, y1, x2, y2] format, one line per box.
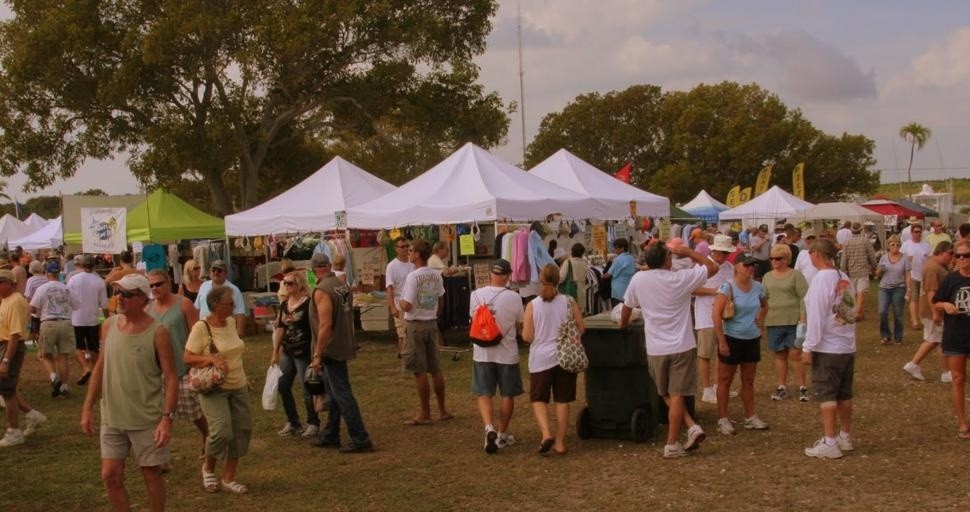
[939, 370, 955, 383]
[701, 383, 739, 404]
[716, 416, 735, 437]
[51, 378, 69, 399]
[279, 421, 372, 454]
[0, 408, 47, 448]
[663, 425, 705, 459]
[904, 361, 925, 382]
[79, 369, 91, 384]
[771, 386, 810, 402]
[484, 424, 515, 454]
[742, 414, 770, 430]
[804, 431, 855, 460]
[880, 337, 903, 345]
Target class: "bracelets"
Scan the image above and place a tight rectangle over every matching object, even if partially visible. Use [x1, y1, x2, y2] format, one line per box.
[3, 358, 9, 363]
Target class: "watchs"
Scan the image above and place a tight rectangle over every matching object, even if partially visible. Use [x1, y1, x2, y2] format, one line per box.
[163, 412, 176, 420]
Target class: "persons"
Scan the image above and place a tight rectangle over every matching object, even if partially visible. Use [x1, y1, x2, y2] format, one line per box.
[804, 239, 857, 459]
[1, 246, 146, 446]
[144, 261, 247, 459]
[825, 218, 970, 382]
[470, 259, 525, 454]
[690, 223, 819, 434]
[620, 240, 720, 457]
[272, 253, 374, 452]
[184, 287, 253, 493]
[81, 274, 178, 511]
[549, 237, 638, 315]
[385, 236, 455, 424]
[931, 239, 969, 438]
[522, 263, 585, 454]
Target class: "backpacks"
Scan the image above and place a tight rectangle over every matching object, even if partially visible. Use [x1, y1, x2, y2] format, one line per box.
[469, 288, 508, 347]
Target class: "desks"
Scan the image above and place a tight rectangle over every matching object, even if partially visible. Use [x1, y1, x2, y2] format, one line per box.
[353, 303, 392, 332]
[254, 296, 279, 333]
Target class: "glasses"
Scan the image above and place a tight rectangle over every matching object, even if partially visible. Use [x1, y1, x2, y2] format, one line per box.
[890, 243, 900, 247]
[913, 230, 922, 234]
[112, 289, 142, 299]
[807, 237, 815, 240]
[741, 263, 756, 267]
[283, 281, 296, 286]
[768, 256, 784, 261]
[955, 252, 970, 259]
[394, 245, 410, 248]
[149, 281, 164, 288]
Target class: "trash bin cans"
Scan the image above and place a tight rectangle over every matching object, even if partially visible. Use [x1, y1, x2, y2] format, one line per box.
[440, 274, 470, 326]
[576, 310, 659, 442]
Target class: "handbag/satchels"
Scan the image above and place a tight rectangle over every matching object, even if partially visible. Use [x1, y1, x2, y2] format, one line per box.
[188, 319, 229, 393]
[304, 363, 332, 412]
[712, 280, 735, 320]
[562, 258, 578, 302]
[558, 295, 590, 373]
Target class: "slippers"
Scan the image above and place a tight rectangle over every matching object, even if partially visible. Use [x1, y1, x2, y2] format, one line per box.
[958, 429, 970, 442]
[403, 411, 458, 425]
[536, 438, 568, 455]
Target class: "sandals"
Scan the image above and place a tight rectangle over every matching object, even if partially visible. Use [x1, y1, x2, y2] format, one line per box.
[201, 463, 248, 495]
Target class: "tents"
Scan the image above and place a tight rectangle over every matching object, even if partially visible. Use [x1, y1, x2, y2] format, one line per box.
[347, 142, 670, 291]
[862, 192, 939, 252]
[224, 156, 399, 282]
[721, 187, 815, 248]
[678, 188, 733, 230]
[0, 212, 63, 251]
[64, 188, 226, 261]
[804, 202, 883, 245]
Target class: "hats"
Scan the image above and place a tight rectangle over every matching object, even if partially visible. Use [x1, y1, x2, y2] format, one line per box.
[311, 254, 329, 267]
[802, 229, 816, 239]
[74, 255, 95, 266]
[707, 233, 736, 254]
[760, 224, 768, 231]
[0, 270, 17, 284]
[734, 252, 758, 265]
[851, 222, 862, 232]
[651, 227, 705, 250]
[332, 256, 346, 270]
[29, 260, 42, 273]
[491, 258, 513, 275]
[46, 261, 61, 272]
[109, 273, 154, 300]
[906, 215, 921, 224]
[210, 259, 227, 271]
